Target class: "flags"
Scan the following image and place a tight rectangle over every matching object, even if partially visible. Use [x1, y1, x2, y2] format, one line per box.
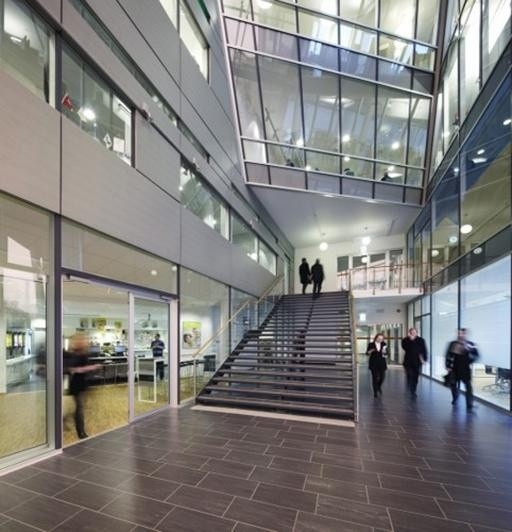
[62, 94, 73, 110]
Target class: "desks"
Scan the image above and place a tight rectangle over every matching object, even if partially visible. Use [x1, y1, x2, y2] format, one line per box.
[84, 355, 215, 404]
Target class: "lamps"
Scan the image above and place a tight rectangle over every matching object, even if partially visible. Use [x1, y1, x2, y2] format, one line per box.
[361, 226, 369, 246]
[317, 232, 328, 252]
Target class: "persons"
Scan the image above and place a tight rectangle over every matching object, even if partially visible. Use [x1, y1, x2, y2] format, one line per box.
[151, 333, 165, 380]
[299, 257, 311, 295]
[184, 333, 193, 346]
[380, 171, 392, 181]
[64, 332, 102, 438]
[366, 334, 390, 398]
[310, 258, 325, 299]
[344, 167, 355, 176]
[443, 328, 480, 409]
[286, 158, 294, 166]
[401, 327, 428, 400]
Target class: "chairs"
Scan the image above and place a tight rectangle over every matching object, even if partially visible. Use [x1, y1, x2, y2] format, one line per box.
[482, 364, 511, 395]
